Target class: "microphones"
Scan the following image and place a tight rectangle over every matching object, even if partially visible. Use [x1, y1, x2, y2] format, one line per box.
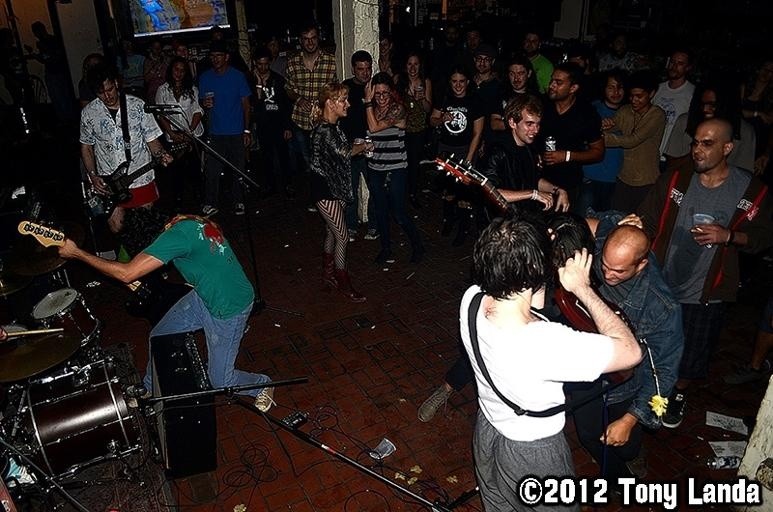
[144, 102, 179, 113]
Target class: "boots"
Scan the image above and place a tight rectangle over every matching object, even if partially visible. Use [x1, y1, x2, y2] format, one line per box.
[321, 252, 340, 288]
[335, 269, 367, 303]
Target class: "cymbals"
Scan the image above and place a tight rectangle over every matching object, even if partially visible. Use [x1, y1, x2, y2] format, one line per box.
[8, 236, 71, 276]
[0, 279, 30, 296]
[0, 330, 83, 382]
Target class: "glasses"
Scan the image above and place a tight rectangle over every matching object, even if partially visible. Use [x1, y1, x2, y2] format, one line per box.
[474, 56, 493, 63]
[374, 91, 391, 97]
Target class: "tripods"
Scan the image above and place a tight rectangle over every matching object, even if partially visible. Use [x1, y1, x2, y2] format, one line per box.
[157, 113, 303, 322]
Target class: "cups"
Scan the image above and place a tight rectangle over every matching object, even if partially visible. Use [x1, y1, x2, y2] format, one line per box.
[205, 91, 215, 108]
[692, 214, 715, 244]
[414, 87, 424, 100]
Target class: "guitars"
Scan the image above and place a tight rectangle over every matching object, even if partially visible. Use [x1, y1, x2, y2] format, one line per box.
[418, 153, 637, 384]
[83, 141, 190, 220]
[16, 220, 194, 326]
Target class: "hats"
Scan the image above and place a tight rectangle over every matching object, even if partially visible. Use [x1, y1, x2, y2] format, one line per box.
[472, 45, 497, 59]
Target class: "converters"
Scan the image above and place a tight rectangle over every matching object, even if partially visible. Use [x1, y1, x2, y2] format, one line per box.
[281, 412, 306, 429]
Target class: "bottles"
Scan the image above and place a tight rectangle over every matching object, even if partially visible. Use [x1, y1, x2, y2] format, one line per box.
[707, 455, 742, 471]
[353, 136, 373, 158]
[546, 135, 556, 152]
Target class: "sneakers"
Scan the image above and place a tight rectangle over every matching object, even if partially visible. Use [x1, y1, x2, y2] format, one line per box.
[233, 202, 245, 215]
[126, 390, 154, 409]
[373, 247, 393, 263]
[660, 386, 689, 428]
[363, 228, 381, 240]
[408, 242, 425, 266]
[346, 227, 358, 243]
[197, 202, 219, 219]
[416, 383, 453, 422]
[254, 386, 278, 413]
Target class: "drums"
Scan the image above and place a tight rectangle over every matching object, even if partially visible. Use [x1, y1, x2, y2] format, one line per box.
[31, 288, 102, 346]
[28, 354, 141, 479]
